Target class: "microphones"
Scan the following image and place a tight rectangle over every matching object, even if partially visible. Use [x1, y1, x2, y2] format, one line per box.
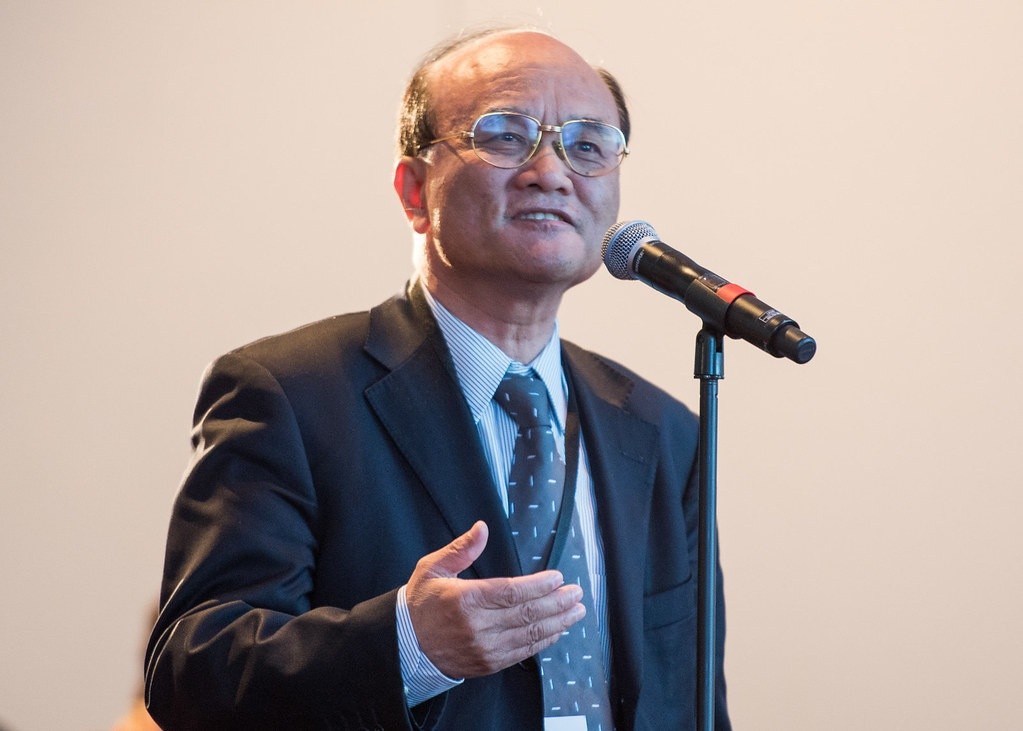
[604, 222, 816, 364]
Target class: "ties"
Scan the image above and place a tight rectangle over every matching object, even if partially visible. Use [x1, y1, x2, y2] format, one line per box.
[495, 374, 617, 731]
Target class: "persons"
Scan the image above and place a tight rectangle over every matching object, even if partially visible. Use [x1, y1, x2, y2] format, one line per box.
[140, 27, 737, 728]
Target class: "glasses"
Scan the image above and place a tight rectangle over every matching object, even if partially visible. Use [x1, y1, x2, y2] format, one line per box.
[414, 112, 632, 178]
